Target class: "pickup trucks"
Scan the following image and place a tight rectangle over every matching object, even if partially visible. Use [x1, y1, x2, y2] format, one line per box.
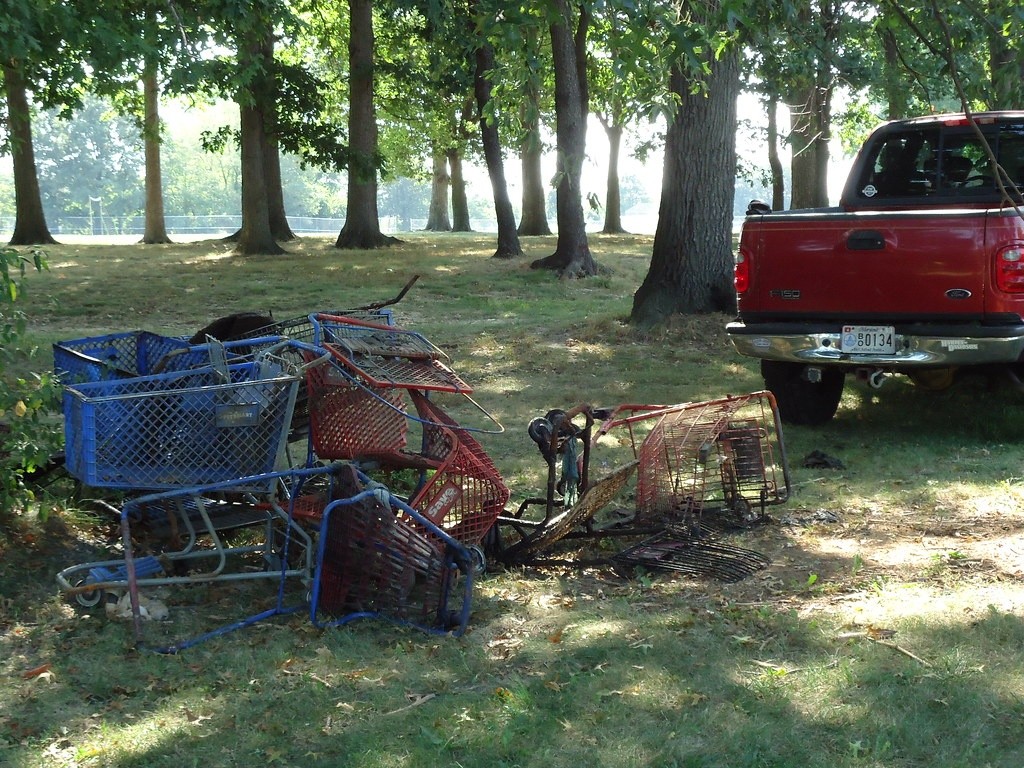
[726, 110, 1024, 430]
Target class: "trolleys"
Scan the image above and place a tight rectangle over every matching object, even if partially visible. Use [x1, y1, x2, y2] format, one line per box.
[49, 272, 791, 661]
